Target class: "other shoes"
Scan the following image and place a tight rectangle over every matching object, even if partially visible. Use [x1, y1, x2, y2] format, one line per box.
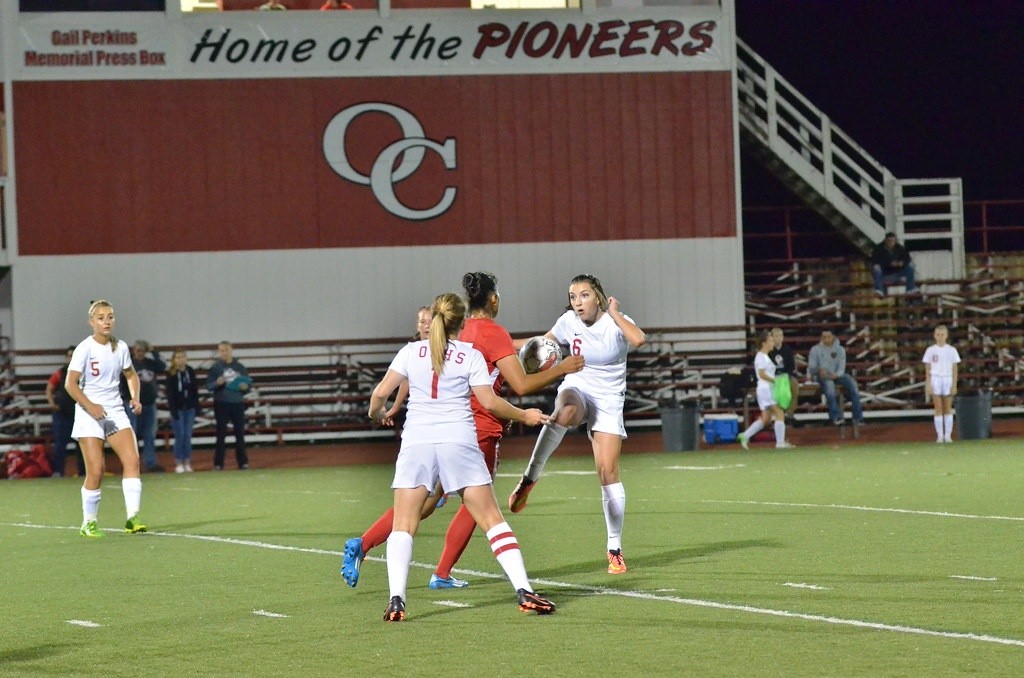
[151, 465, 165, 473]
[834, 418, 845, 426]
[185, 465, 193, 472]
[906, 288, 920, 294]
[212, 465, 223, 471]
[241, 463, 248, 470]
[854, 419, 865, 426]
[176, 465, 185, 474]
[52, 472, 61, 477]
[876, 290, 882, 297]
[786, 417, 804, 429]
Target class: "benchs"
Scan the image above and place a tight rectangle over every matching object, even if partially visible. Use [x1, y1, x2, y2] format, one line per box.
[0, 253, 1024, 455]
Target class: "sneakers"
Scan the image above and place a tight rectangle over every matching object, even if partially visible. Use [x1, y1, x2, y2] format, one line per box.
[508, 475, 537, 513]
[515, 589, 556, 614]
[79, 521, 103, 537]
[430, 574, 469, 589]
[341, 537, 367, 587]
[776, 441, 795, 448]
[739, 433, 749, 450]
[383, 596, 405, 621]
[125, 517, 147, 534]
[607, 548, 627, 574]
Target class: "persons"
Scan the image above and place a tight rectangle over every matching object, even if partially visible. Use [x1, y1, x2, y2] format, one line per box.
[65, 300, 146, 537]
[511, 274, 645, 573]
[921, 325, 960, 443]
[207, 341, 253, 471]
[258, 0, 285, 10]
[166, 348, 200, 474]
[46, 346, 85, 478]
[768, 328, 801, 426]
[871, 232, 920, 297]
[319, 0, 352, 9]
[417, 306, 449, 507]
[120, 339, 166, 471]
[808, 329, 867, 425]
[738, 327, 797, 449]
[369, 293, 556, 621]
[340, 273, 585, 589]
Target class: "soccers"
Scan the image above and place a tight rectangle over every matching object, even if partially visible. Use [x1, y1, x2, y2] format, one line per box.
[518, 335, 564, 375]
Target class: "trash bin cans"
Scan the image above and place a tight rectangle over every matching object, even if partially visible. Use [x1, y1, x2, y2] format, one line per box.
[952, 387, 992, 440]
[657, 397, 701, 453]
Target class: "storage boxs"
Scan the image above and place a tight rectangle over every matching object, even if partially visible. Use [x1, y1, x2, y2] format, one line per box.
[702, 413, 739, 445]
